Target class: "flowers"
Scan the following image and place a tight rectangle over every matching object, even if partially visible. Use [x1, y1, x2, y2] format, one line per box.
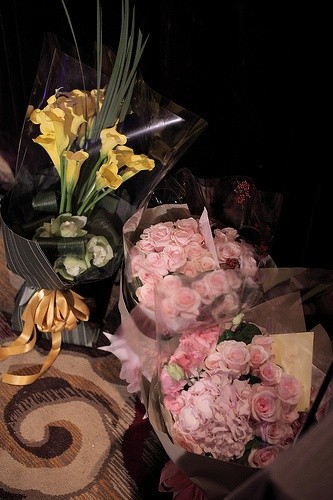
[160, 328, 302, 469]
[30, 0, 157, 283]
[124, 181, 276, 333]
